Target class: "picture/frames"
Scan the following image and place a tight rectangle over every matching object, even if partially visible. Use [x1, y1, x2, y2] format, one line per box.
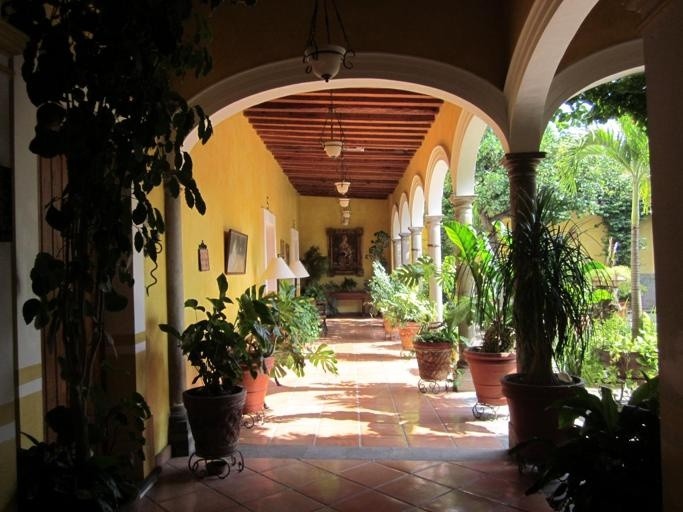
[323, 226, 363, 278]
[223, 228, 248, 276]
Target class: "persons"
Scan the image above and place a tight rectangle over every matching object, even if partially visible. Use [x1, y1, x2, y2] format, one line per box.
[338, 234, 350, 266]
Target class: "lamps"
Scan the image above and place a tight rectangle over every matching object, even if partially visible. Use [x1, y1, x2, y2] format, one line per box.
[301, 1, 356, 83]
[318, 91, 353, 227]
[260, 252, 310, 358]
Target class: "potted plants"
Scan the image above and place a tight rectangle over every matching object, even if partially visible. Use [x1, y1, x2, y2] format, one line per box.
[157, 273, 341, 479]
[361, 230, 391, 318]
[443, 219, 616, 406]
[451, 181, 616, 439]
[374, 256, 493, 382]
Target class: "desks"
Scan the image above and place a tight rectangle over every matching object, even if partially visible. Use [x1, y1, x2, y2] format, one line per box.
[326, 290, 368, 318]
